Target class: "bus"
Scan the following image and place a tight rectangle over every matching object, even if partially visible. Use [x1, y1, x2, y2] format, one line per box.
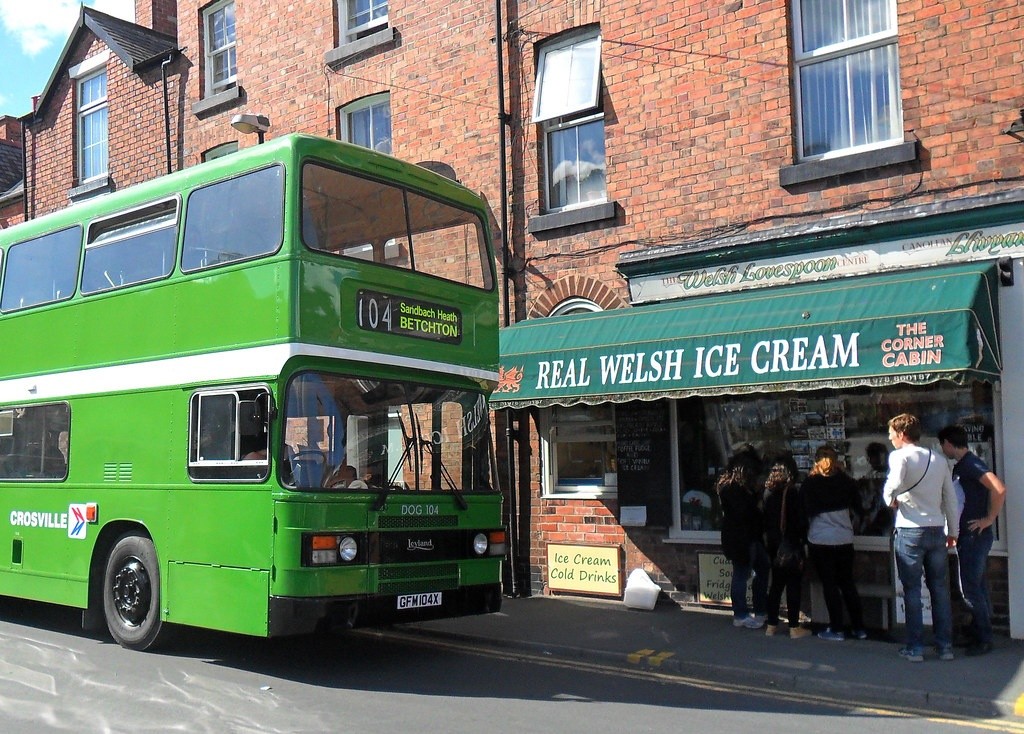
[0, 131, 507, 651]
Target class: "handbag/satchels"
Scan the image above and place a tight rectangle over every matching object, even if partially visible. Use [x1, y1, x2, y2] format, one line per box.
[774, 535, 807, 577]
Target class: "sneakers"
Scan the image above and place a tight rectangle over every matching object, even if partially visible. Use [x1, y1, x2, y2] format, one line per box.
[733, 616, 763, 629]
[818, 631, 846, 641]
[766, 621, 789, 636]
[935, 648, 954, 660]
[756, 614, 768, 625]
[789, 624, 812, 639]
[899, 648, 924, 662]
[851, 631, 867, 639]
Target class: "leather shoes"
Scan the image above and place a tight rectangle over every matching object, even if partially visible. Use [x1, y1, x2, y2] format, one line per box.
[965, 638, 993, 656]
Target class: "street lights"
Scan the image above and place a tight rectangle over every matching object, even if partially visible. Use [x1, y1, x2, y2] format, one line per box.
[231, 114, 271, 145]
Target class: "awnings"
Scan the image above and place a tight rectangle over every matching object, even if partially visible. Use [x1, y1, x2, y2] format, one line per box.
[488, 259, 1002, 410]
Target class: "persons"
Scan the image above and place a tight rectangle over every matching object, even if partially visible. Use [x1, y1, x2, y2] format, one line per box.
[242, 444, 267, 460]
[937, 425, 1006, 654]
[859, 443, 897, 536]
[764, 456, 812, 639]
[802, 446, 868, 641]
[882, 414, 958, 661]
[717, 457, 768, 629]
